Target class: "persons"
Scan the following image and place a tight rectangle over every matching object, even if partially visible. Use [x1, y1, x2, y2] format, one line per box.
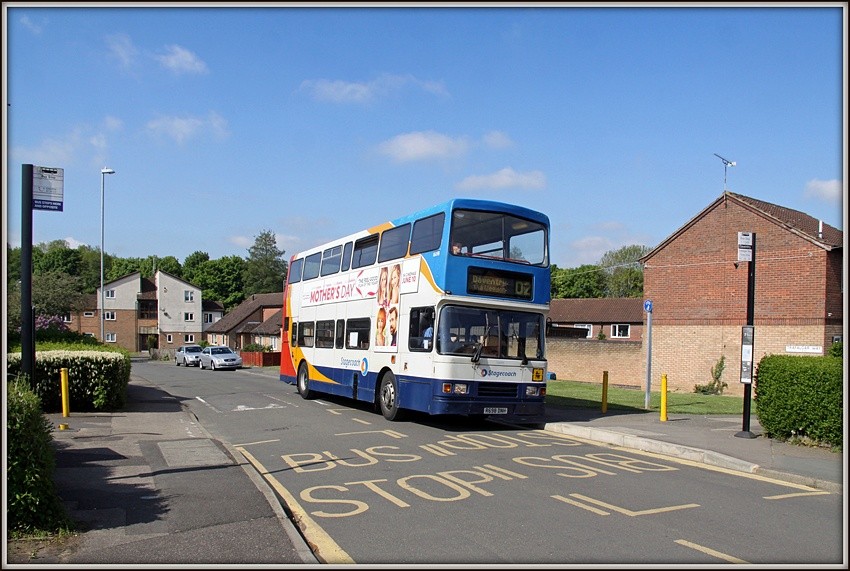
[423, 325, 433, 348]
[387, 263, 401, 303]
[450, 240, 462, 257]
[375, 307, 386, 345]
[377, 267, 389, 307]
[386, 305, 398, 346]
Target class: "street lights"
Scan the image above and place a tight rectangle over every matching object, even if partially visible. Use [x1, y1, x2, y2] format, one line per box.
[100, 166, 116, 346]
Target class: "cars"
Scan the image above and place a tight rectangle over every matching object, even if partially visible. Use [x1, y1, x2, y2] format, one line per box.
[199, 346, 242, 371]
[174, 345, 203, 367]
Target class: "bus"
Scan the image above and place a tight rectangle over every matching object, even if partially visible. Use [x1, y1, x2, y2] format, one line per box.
[279, 197, 551, 425]
